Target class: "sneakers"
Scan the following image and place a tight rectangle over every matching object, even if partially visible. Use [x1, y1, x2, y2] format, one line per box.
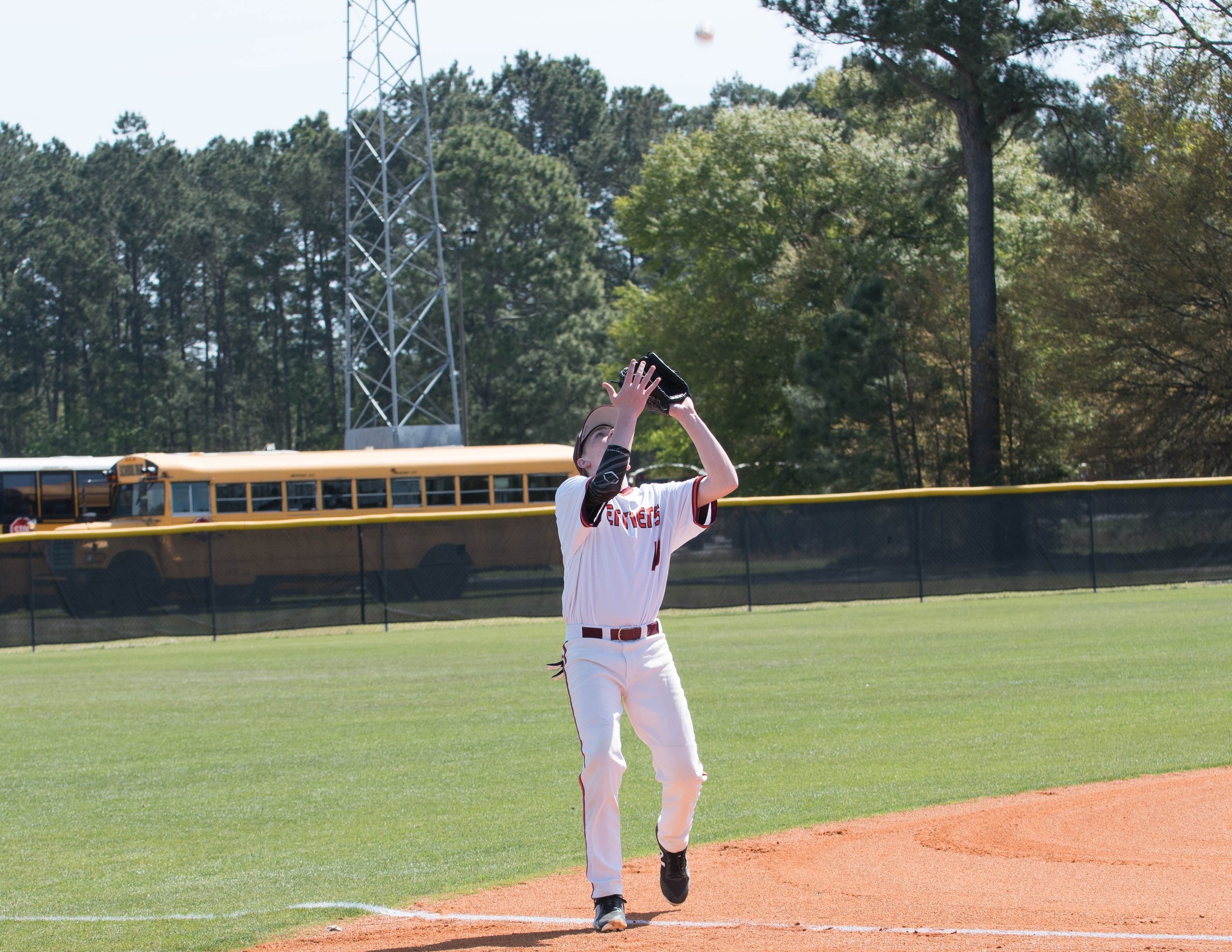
[655, 823, 690, 907]
[592, 893, 628, 932]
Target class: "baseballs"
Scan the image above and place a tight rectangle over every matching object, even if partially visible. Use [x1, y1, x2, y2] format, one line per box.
[694, 25, 715, 38]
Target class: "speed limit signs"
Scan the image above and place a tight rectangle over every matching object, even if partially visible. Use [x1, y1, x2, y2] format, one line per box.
[10, 517, 34, 533]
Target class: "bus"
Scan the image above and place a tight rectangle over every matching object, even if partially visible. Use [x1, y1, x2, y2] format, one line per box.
[0, 456, 130, 613]
[43, 443, 584, 619]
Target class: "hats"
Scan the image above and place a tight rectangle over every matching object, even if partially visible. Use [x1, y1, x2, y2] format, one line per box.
[572, 405, 614, 475]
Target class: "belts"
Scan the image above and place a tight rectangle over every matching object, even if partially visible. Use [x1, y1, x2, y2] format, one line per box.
[583, 621, 659, 641]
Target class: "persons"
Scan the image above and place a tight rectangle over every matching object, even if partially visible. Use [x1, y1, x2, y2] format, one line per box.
[553, 352, 737, 930]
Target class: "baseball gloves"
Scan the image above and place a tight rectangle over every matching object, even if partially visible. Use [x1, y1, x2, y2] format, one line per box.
[618, 352, 691, 413]
